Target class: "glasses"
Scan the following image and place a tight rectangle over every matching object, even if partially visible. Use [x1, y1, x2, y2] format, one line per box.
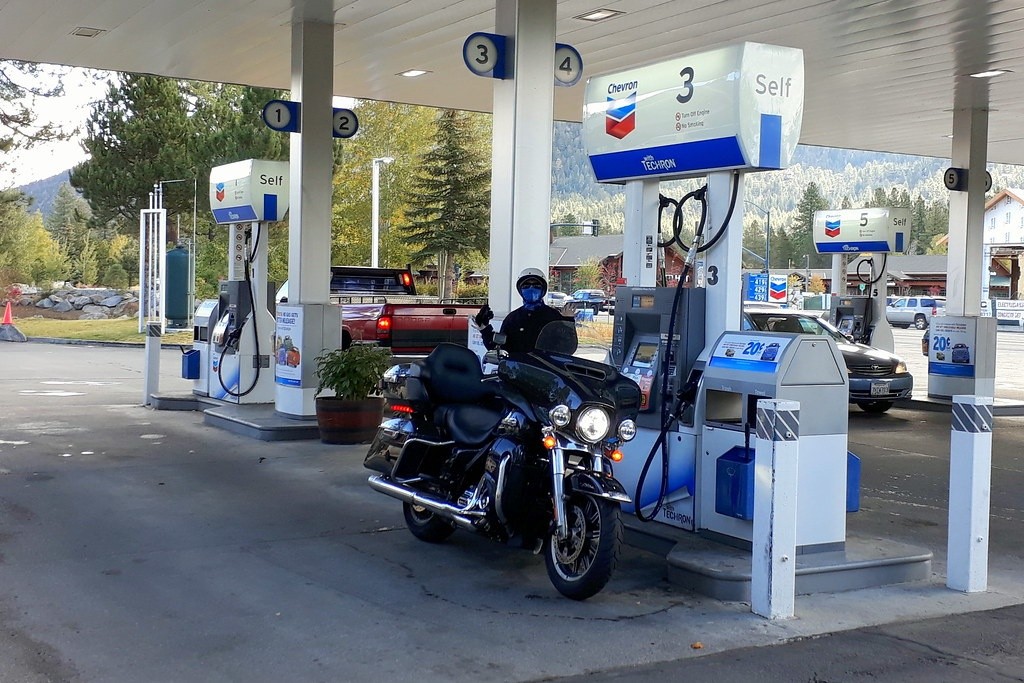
[520, 281, 543, 290]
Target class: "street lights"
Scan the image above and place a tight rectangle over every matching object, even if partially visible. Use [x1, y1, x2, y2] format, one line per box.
[370, 156, 394, 267]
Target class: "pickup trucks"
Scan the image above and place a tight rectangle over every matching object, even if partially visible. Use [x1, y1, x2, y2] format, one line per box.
[565, 292, 616, 317]
[328, 265, 489, 353]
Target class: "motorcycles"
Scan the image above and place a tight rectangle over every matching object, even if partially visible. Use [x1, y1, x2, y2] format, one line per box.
[363, 331, 639, 601]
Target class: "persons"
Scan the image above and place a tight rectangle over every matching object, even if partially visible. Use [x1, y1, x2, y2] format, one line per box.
[499, 267, 576, 361]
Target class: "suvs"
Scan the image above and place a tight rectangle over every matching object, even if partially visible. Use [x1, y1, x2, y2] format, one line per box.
[884, 295, 949, 331]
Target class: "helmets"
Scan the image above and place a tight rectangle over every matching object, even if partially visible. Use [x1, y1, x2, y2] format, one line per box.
[516, 267, 548, 298]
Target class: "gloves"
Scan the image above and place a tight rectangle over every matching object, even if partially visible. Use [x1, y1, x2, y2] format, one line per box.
[475, 304, 494, 327]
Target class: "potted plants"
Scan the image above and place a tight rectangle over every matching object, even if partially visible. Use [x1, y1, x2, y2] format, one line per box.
[312, 341, 396, 444]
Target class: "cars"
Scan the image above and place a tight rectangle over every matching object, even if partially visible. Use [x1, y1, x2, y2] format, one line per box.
[743, 299, 782, 311]
[740, 310, 914, 412]
[548, 288, 606, 312]
